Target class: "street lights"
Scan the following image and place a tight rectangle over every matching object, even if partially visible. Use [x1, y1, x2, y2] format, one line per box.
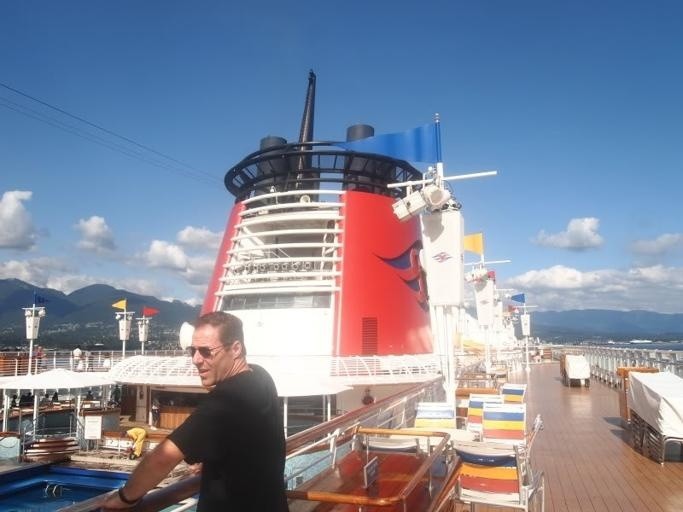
[22, 303, 45, 375]
[115, 311, 153, 360]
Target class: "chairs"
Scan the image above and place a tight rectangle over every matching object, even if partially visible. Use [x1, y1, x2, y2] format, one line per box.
[358, 383, 546, 510]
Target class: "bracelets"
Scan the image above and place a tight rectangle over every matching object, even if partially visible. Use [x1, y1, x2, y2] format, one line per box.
[118, 487, 142, 504]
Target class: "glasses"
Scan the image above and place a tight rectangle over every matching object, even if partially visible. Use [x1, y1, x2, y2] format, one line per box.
[186, 344, 233, 358]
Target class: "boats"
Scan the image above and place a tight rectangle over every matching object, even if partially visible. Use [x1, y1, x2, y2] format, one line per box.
[629, 339, 652, 344]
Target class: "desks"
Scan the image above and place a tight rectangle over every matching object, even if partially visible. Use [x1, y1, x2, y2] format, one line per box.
[279, 426, 462, 511]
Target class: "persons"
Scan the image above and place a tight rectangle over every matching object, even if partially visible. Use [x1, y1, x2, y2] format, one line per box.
[102, 352, 112, 371]
[19, 393, 34, 407]
[55, 312, 290, 512]
[31, 345, 47, 375]
[52, 392, 59, 403]
[119, 427, 146, 461]
[110, 384, 120, 404]
[151, 394, 161, 426]
[73, 344, 95, 372]
[41, 394, 51, 407]
[362, 387, 374, 406]
[85, 391, 93, 400]
[10, 395, 17, 409]
